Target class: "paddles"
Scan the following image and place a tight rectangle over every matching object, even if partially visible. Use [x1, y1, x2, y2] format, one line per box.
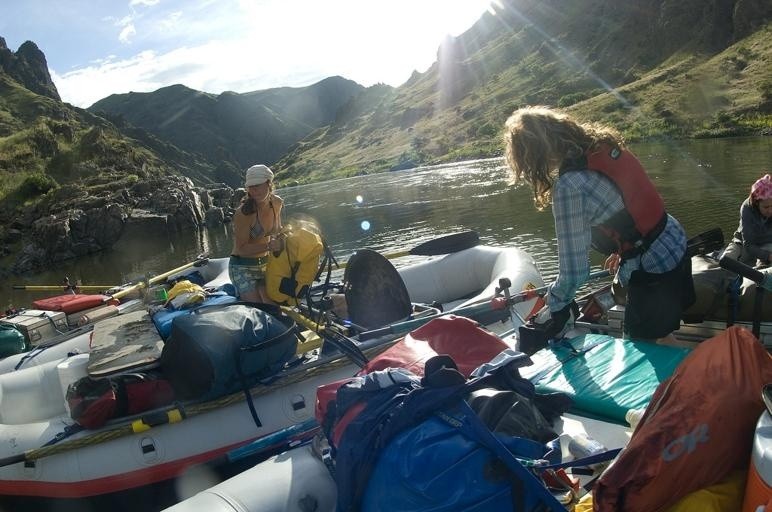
[360, 227, 723, 341]
[321, 232, 479, 273]
[280, 305, 371, 368]
[1, 295, 508, 511]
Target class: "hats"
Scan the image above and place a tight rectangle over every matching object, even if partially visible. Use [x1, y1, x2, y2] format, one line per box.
[244, 164, 275, 189]
[750, 174, 772, 199]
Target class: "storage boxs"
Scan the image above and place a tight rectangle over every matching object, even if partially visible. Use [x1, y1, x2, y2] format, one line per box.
[0, 308, 68, 346]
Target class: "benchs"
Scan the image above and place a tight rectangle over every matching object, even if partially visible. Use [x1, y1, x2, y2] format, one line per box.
[88, 309, 164, 382]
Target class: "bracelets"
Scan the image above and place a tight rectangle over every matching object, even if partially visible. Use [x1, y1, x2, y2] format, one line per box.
[264, 241, 271, 252]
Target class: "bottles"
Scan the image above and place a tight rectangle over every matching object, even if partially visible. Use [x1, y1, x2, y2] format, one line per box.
[568, 432, 615, 474]
[624, 408, 647, 434]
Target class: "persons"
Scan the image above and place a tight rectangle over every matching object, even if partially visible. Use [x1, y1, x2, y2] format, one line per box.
[718, 174, 772, 271]
[500, 102, 698, 350]
[228, 163, 285, 314]
